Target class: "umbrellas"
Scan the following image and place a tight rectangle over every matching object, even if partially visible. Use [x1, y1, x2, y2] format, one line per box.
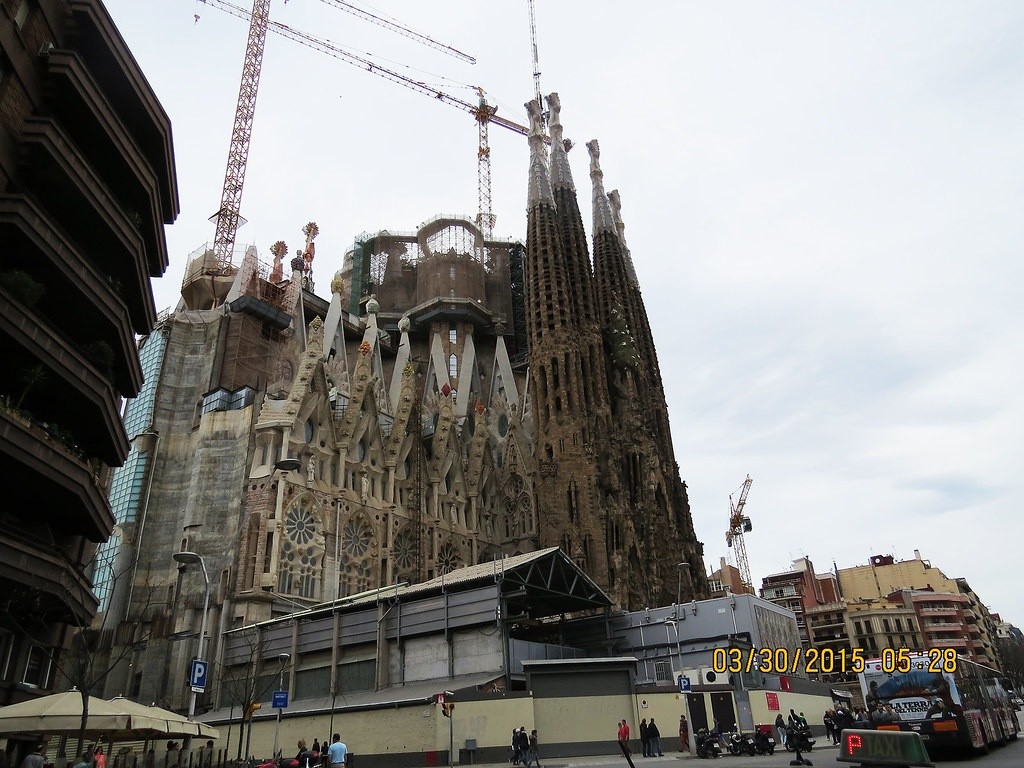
[0, 685, 219, 760]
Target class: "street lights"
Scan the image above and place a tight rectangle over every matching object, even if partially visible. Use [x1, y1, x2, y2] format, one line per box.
[272, 653, 290, 760]
[664, 562, 697, 754]
[173, 551, 211, 750]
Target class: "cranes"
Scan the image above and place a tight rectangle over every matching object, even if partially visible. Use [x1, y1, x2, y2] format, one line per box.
[725, 474, 753, 594]
[195, 0, 574, 275]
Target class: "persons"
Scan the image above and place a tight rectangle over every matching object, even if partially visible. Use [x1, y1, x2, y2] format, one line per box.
[73, 744, 107, 768]
[173, 742, 181, 751]
[327, 734, 348, 768]
[21, 745, 44, 768]
[921, 672, 955, 719]
[799, 712, 807, 727]
[866, 680, 901, 722]
[648, 718, 664, 757]
[678, 715, 690, 752]
[295, 737, 329, 764]
[509, 726, 544, 768]
[712, 717, 728, 746]
[167, 741, 178, 751]
[640, 718, 651, 757]
[618, 719, 633, 757]
[207, 740, 214, 748]
[775, 714, 786, 747]
[823, 705, 869, 745]
[788, 709, 801, 728]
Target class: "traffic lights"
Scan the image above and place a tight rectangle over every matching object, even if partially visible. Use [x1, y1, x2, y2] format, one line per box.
[441, 703, 449, 716]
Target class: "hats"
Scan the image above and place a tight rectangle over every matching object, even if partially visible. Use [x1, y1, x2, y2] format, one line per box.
[516, 729, 520, 732]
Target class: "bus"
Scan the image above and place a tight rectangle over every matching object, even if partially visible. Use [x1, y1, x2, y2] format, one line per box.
[855, 650, 1024, 756]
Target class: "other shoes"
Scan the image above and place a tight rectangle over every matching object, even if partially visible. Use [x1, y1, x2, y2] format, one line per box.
[629, 753, 632, 756]
[652, 755, 657, 757]
[643, 756, 647, 757]
[648, 755, 652, 757]
[679, 750, 683, 752]
[828, 738, 829, 741]
[833, 742, 836, 745]
[659, 754, 664, 757]
[508, 758, 544, 768]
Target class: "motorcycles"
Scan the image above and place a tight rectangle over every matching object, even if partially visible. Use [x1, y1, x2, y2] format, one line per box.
[785, 728, 813, 752]
[727, 723, 776, 756]
[697, 728, 723, 759]
